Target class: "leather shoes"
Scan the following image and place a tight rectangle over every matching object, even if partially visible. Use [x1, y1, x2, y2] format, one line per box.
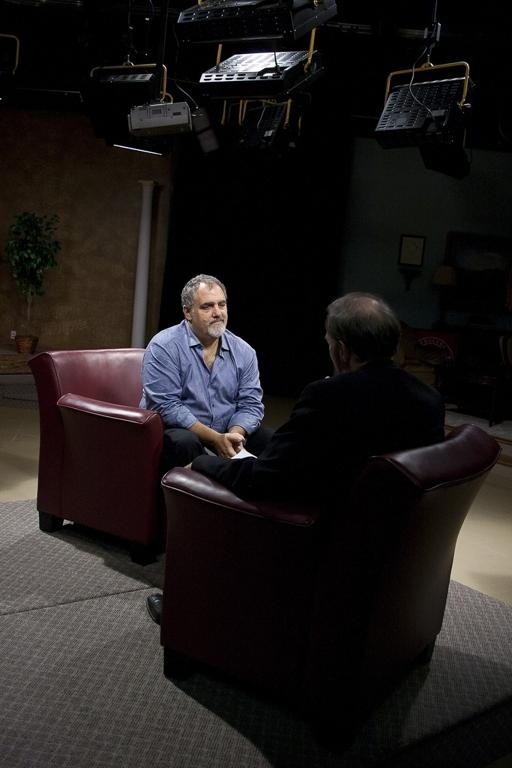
[145, 592, 162, 623]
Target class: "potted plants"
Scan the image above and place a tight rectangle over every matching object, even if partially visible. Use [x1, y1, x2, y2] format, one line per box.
[0, 211, 63, 355]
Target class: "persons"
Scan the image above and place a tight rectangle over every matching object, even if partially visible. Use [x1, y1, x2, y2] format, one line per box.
[138, 273, 277, 576]
[145, 289, 446, 629]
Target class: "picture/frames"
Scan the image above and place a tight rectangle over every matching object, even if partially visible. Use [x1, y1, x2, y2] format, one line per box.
[397, 234, 427, 266]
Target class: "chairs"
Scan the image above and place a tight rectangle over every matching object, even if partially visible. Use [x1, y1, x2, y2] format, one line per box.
[160, 423, 503, 755]
[27, 347, 172, 566]
